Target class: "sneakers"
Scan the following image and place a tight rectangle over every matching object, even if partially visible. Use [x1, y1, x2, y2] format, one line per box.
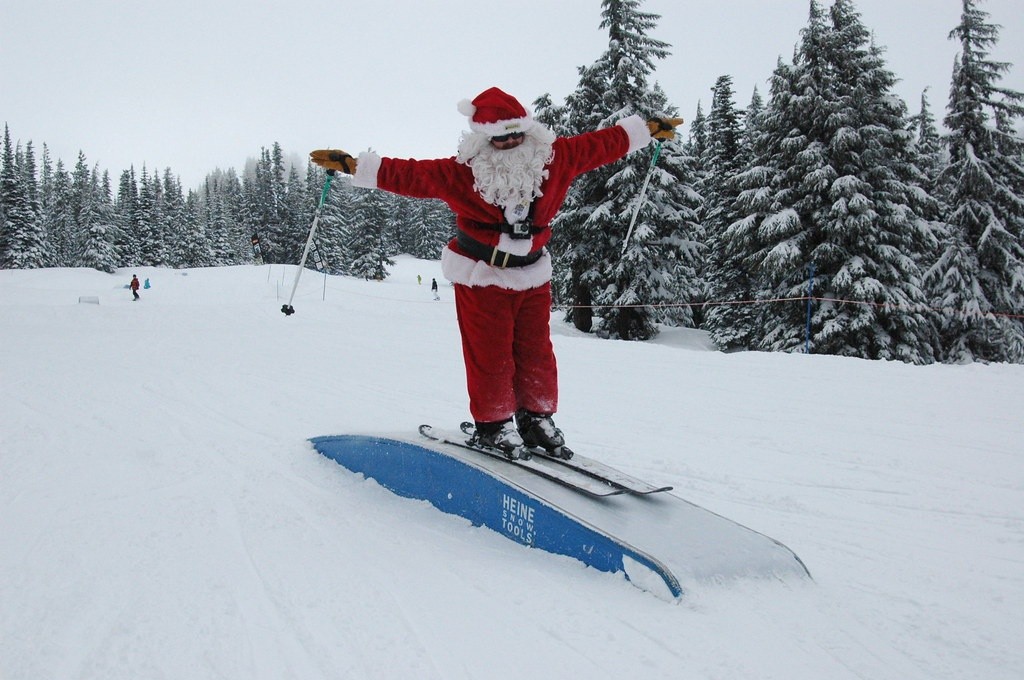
[478, 421, 523, 449]
[520, 413, 567, 449]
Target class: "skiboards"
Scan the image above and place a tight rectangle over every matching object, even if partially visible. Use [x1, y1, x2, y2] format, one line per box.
[461, 421, 673, 497]
[418, 421, 624, 501]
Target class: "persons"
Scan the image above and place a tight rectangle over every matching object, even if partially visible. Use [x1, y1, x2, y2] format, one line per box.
[309, 86, 683, 460]
[431, 278, 440, 300]
[130, 274, 139, 301]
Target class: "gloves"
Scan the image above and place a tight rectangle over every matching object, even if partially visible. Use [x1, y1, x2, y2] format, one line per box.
[647, 117, 684, 140]
[309, 148, 358, 176]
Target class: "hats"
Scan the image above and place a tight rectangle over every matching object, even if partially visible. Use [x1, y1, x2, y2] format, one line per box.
[455, 86, 533, 138]
